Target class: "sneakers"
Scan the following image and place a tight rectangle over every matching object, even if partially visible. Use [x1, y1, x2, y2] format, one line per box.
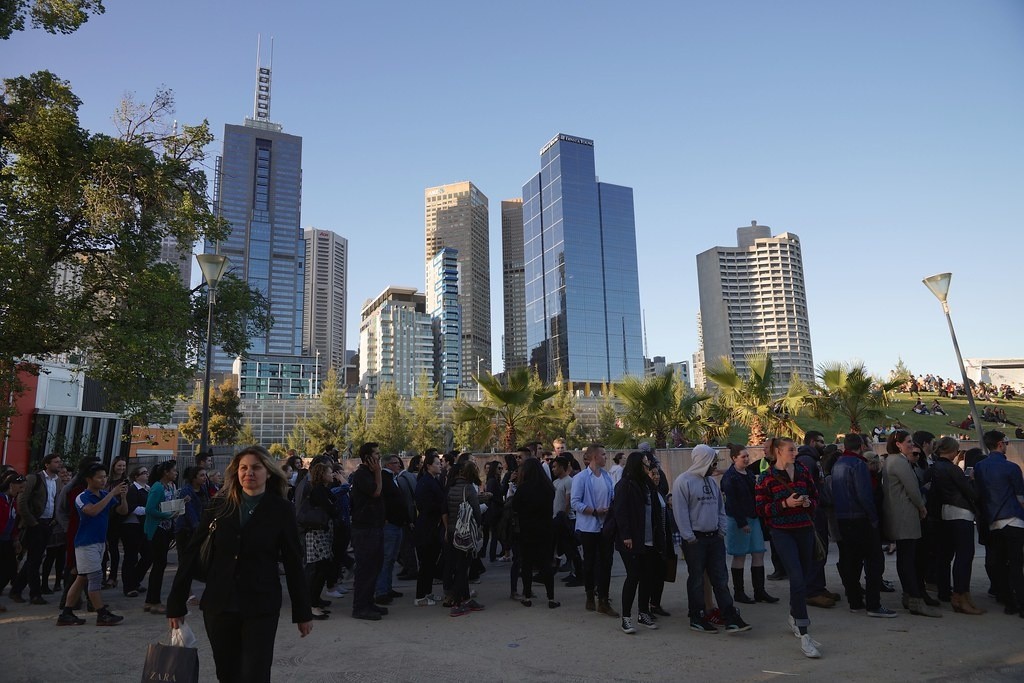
[866, 606, 898, 618]
[688, 606, 752, 633]
[621, 617, 637, 633]
[787, 616, 820, 658]
[849, 602, 865, 612]
[637, 612, 658, 629]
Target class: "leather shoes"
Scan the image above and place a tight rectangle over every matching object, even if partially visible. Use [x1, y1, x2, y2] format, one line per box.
[902, 592, 912, 609]
[908, 598, 942, 618]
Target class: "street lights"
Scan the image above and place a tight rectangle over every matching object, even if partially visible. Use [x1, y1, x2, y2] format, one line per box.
[196, 253, 230, 453]
[922, 272, 989, 455]
[478, 359, 484, 401]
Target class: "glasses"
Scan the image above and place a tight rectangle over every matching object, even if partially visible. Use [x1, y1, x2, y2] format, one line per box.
[516, 455, 522, 459]
[814, 439, 824, 444]
[641, 461, 651, 466]
[895, 429, 903, 439]
[911, 452, 922, 457]
[1002, 441, 1008, 445]
[139, 471, 148, 476]
[497, 467, 503, 470]
[711, 462, 717, 466]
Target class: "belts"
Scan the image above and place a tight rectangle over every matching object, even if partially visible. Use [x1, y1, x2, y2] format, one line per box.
[695, 531, 718, 536]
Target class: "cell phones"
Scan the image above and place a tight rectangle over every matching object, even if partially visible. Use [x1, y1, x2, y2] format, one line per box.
[364, 454, 372, 464]
[798, 495, 809, 501]
[123, 479, 128, 486]
[965, 467, 973, 476]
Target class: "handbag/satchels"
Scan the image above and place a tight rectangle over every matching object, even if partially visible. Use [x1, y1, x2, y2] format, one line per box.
[297, 487, 330, 529]
[453, 482, 478, 550]
[602, 475, 646, 542]
[141, 627, 199, 683]
[662, 553, 679, 583]
[192, 503, 219, 583]
[160, 498, 186, 516]
[813, 531, 827, 562]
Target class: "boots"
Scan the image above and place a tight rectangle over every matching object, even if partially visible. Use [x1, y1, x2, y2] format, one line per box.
[732, 566, 779, 603]
[951, 593, 984, 615]
[586, 589, 620, 617]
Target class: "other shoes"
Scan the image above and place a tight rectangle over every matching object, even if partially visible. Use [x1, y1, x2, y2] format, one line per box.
[57, 614, 86, 625]
[0, 561, 200, 615]
[988, 586, 1024, 618]
[509, 559, 584, 609]
[646, 606, 671, 619]
[396, 547, 514, 585]
[767, 544, 972, 606]
[96, 611, 123, 626]
[312, 563, 485, 620]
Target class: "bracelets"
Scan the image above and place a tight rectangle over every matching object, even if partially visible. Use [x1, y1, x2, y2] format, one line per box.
[592, 509, 597, 517]
[784, 498, 789, 509]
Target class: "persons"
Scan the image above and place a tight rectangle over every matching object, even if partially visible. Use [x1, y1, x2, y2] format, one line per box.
[0, 374, 1024, 657]
[166, 445, 313, 683]
